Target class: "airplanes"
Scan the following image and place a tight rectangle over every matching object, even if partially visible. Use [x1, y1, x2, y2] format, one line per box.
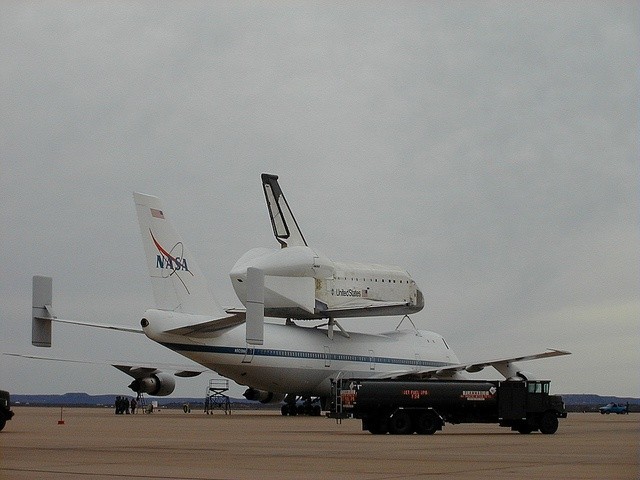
[221, 173, 426, 318]
[30, 171, 573, 419]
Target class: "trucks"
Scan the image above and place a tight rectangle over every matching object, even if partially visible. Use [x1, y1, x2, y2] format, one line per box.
[0, 389, 14, 432]
[353, 373, 568, 436]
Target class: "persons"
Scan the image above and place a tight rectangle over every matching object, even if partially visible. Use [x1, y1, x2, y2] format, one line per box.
[113, 396, 135, 416]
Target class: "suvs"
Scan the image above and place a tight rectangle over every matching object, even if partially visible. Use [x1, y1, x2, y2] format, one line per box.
[599, 402, 627, 415]
